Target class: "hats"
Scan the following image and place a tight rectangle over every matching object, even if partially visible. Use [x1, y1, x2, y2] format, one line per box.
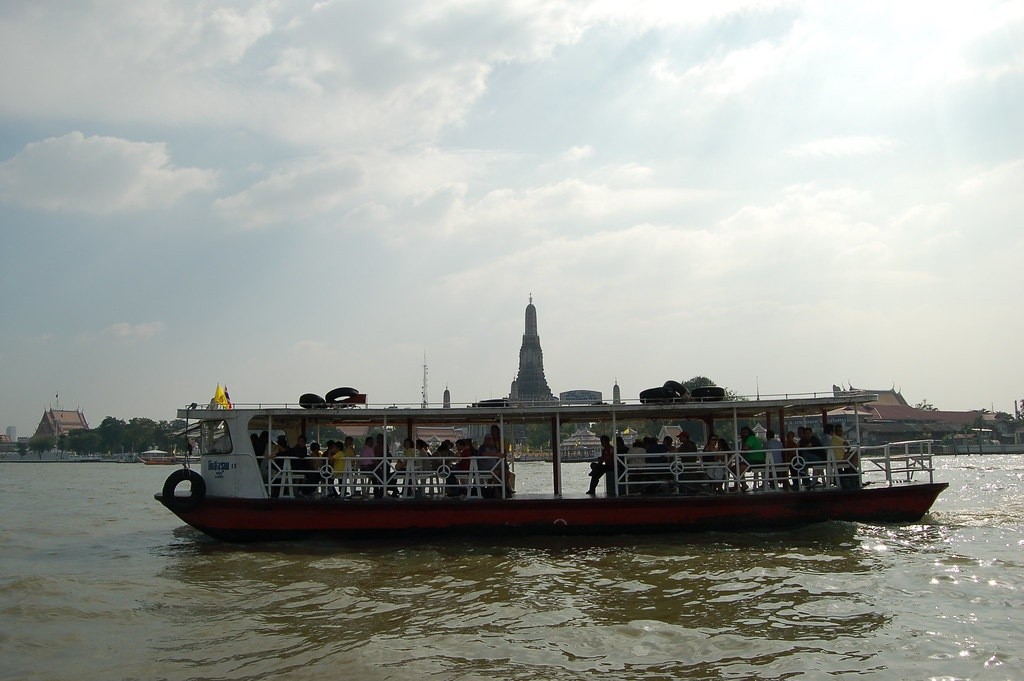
[676, 431, 689, 437]
[485, 434, 492, 438]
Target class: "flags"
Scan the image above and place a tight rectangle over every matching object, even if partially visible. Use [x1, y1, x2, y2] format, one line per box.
[225, 386, 231, 408]
[214, 385, 229, 410]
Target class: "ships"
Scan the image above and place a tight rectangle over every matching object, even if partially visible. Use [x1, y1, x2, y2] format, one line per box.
[154, 380, 950, 543]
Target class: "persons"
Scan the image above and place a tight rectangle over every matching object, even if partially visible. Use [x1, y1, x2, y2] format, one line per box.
[586, 424, 867, 495]
[212, 424, 516, 499]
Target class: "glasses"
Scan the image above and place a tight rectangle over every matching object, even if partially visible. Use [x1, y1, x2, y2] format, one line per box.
[711, 438, 718, 441]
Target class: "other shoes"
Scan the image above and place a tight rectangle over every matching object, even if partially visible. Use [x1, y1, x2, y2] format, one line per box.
[728, 487, 737, 492]
[589, 470, 599, 476]
[740, 484, 749, 491]
[586, 491, 595, 494]
